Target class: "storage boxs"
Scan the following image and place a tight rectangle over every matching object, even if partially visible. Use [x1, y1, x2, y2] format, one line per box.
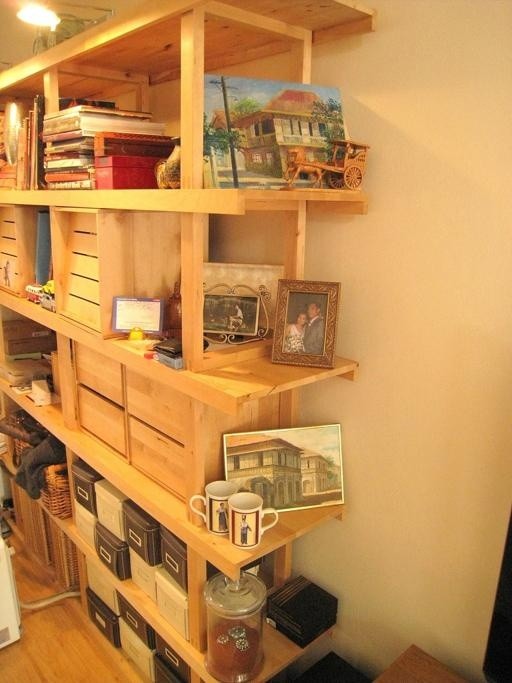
[4, 337, 57, 355]
[2, 320, 53, 341]
[93, 155, 164, 189]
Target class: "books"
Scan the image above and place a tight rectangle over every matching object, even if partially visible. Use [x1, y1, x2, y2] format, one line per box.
[7, 335, 57, 355]
[15, 93, 116, 190]
[43, 352, 52, 362]
[42, 104, 167, 189]
[0, 109, 16, 191]
[2, 318, 56, 353]
[36, 210, 51, 284]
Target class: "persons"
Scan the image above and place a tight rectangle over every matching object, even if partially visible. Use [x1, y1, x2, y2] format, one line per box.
[227, 304, 244, 329]
[302, 301, 324, 354]
[216, 502, 229, 531]
[283, 311, 308, 354]
[239, 518, 252, 544]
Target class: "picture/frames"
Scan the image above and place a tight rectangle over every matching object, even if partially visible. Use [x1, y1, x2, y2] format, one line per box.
[111, 297, 166, 334]
[202, 283, 272, 346]
[203, 261, 285, 329]
[223, 423, 344, 514]
[271, 278, 340, 369]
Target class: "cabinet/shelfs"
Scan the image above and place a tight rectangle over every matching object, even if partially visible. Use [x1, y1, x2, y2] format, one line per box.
[0, 0, 376, 682]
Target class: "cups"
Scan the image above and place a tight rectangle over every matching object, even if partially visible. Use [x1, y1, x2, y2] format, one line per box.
[226, 491, 279, 548]
[189, 479, 239, 536]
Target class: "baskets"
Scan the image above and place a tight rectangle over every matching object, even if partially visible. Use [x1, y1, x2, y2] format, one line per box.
[14, 438, 72, 520]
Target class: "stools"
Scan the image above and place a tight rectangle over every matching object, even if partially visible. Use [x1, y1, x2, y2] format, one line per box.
[374, 638, 473, 682]
